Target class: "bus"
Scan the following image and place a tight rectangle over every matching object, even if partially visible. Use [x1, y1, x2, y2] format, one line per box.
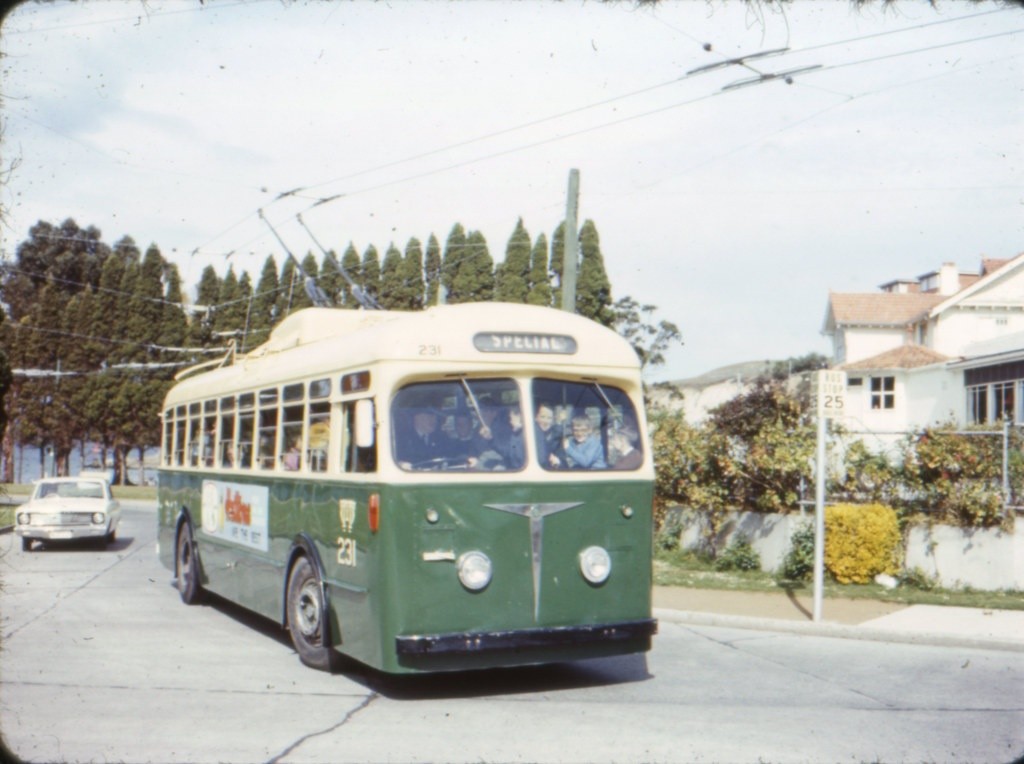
[155, 305, 660, 673]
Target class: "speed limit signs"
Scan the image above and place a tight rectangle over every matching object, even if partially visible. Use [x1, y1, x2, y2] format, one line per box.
[818, 369, 848, 420]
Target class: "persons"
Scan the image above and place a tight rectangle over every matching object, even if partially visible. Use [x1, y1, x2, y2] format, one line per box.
[444, 410, 476, 455]
[561, 412, 606, 469]
[479, 405, 526, 470]
[398, 401, 478, 470]
[536, 403, 569, 470]
[611, 426, 643, 471]
[472, 397, 498, 434]
[189, 415, 329, 471]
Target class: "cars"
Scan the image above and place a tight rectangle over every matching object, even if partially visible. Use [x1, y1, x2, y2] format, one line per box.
[16, 476, 122, 550]
